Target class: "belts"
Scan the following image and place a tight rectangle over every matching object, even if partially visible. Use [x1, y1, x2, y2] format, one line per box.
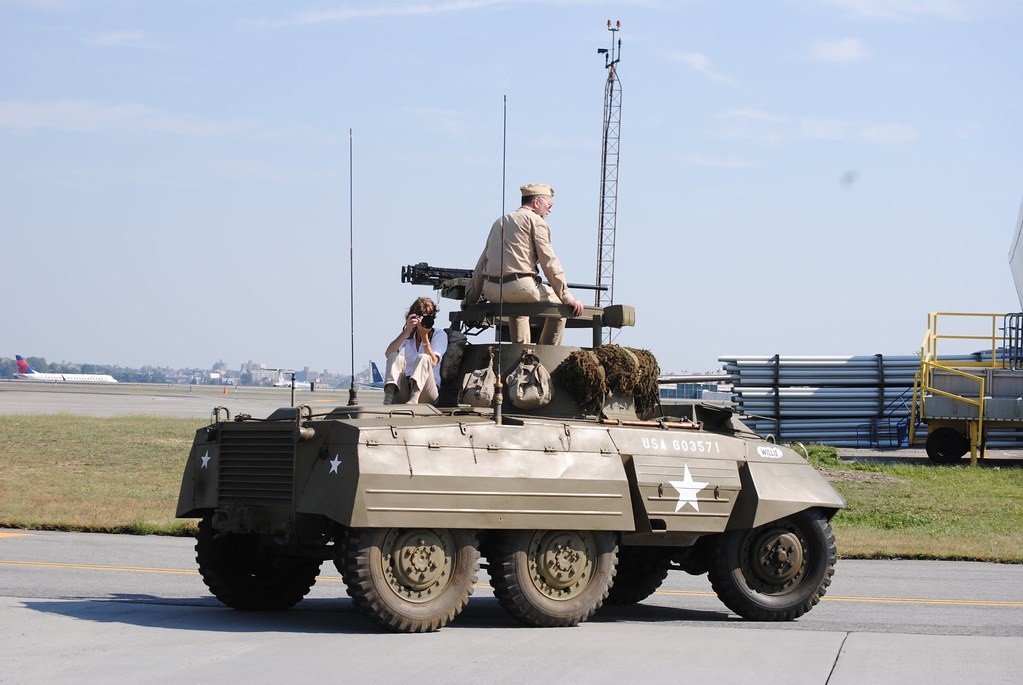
[482, 272, 532, 284]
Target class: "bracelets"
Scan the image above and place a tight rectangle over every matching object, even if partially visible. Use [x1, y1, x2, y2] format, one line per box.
[421, 340, 430, 345]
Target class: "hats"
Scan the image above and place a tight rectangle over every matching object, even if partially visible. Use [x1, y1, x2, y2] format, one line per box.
[520, 183, 555, 198]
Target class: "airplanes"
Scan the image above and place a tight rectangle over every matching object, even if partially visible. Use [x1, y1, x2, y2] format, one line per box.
[366, 359, 384, 391]
[13, 354, 118, 385]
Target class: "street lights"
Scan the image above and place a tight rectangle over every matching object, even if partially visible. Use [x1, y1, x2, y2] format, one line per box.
[284, 372, 295, 407]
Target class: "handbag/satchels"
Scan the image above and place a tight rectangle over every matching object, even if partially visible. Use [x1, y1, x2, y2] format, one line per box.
[439, 326, 466, 384]
[506, 352, 555, 409]
[456, 367, 496, 407]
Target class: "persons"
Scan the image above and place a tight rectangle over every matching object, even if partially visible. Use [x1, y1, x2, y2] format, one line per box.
[379, 296, 448, 407]
[458, 182, 584, 346]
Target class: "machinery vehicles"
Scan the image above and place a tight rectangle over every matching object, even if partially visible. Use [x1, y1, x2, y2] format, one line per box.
[176, 13, 847, 632]
[907, 310, 1023, 467]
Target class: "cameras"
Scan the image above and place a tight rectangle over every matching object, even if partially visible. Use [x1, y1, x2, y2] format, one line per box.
[415, 313, 434, 328]
